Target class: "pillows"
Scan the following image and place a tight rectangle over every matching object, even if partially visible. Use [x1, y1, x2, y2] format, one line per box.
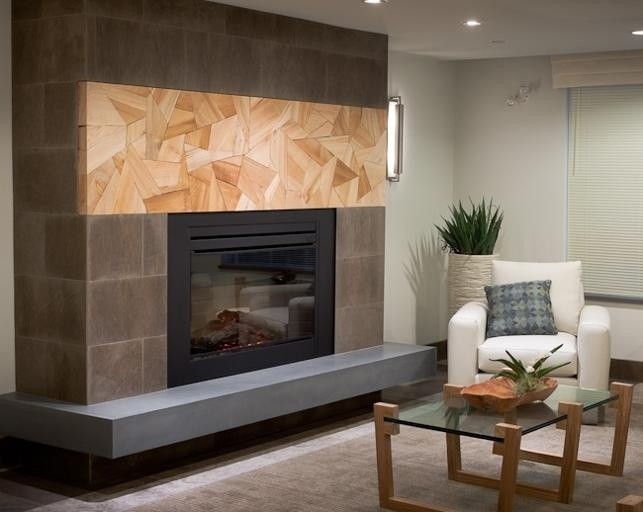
[484, 280, 559, 339]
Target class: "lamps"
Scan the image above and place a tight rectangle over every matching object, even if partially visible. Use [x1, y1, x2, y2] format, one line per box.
[386, 95, 404, 182]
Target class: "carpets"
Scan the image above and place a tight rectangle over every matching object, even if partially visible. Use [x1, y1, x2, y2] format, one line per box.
[0, 358, 642, 512]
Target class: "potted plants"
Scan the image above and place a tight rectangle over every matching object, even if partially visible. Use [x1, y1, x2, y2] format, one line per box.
[432, 195, 505, 319]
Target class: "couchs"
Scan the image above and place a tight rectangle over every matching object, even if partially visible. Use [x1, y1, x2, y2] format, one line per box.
[447, 259, 612, 418]
